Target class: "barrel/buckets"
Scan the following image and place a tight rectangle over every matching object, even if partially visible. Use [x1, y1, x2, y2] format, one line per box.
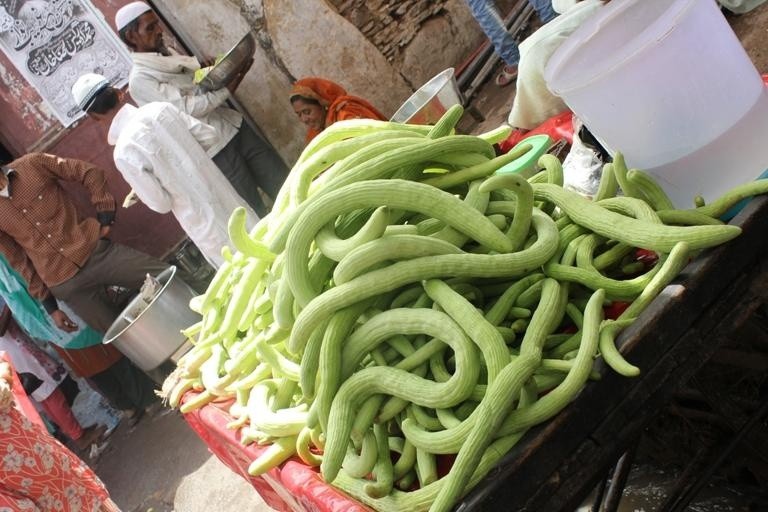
[543, 1, 767, 212]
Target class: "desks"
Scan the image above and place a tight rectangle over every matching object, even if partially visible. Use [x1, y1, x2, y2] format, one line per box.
[176, 110, 768, 511]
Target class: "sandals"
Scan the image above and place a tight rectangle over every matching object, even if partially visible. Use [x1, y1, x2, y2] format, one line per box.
[496, 64, 518, 86]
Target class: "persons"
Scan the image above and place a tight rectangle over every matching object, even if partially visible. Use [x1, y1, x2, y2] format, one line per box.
[0, 152, 208, 387]
[0, 250, 161, 427]
[289, 76, 388, 148]
[0, 349, 122, 511]
[0, 295, 108, 451]
[112, 0, 289, 219]
[71, 72, 262, 276]
[461, 1, 557, 87]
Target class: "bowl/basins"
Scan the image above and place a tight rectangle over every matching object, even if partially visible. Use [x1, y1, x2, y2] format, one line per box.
[389, 68, 461, 126]
[196, 31, 254, 92]
[102, 265, 198, 370]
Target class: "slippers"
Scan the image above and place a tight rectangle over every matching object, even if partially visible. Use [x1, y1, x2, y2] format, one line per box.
[127, 409, 144, 426]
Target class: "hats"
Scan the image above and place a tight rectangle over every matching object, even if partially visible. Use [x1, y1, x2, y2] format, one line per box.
[71, 74, 109, 110]
[116, 1, 152, 30]
